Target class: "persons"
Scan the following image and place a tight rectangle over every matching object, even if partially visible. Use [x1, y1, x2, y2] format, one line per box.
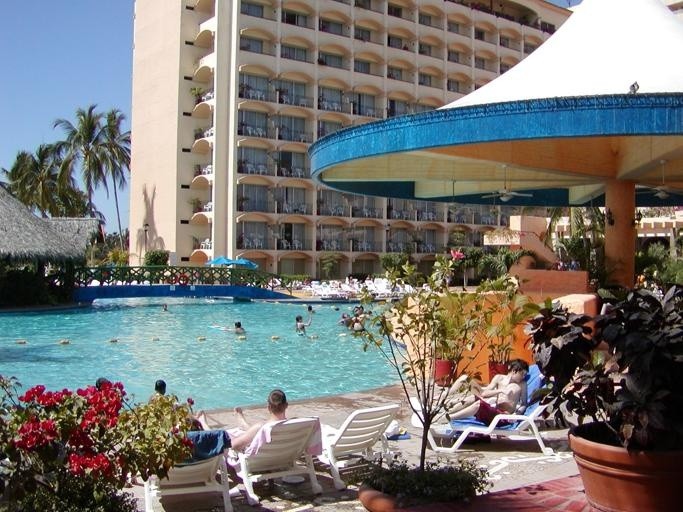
[443, 359, 528, 427]
[339, 306, 372, 337]
[235, 322, 243, 334]
[303, 274, 373, 293]
[296, 306, 315, 336]
[162, 306, 170, 314]
[149, 380, 165, 408]
[96, 377, 110, 395]
[195, 390, 287, 454]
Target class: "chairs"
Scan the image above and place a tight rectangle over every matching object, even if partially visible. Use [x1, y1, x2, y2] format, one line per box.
[228, 418, 323, 507]
[145, 430, 230, 511]
[324, 404, 401, 491]
[412, 376, 556, 454]
[309, 276, 416, 300]
[412, 364, 541, 411]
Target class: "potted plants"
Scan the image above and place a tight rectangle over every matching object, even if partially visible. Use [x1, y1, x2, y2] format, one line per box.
[416, 261, 534, 385]
[358, 462, 483, 511]
[532, 282, 682, 511]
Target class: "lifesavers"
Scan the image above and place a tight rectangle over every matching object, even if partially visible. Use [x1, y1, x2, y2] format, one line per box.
[179, 274, 189, 285]
[168, 275, 178, 284]
